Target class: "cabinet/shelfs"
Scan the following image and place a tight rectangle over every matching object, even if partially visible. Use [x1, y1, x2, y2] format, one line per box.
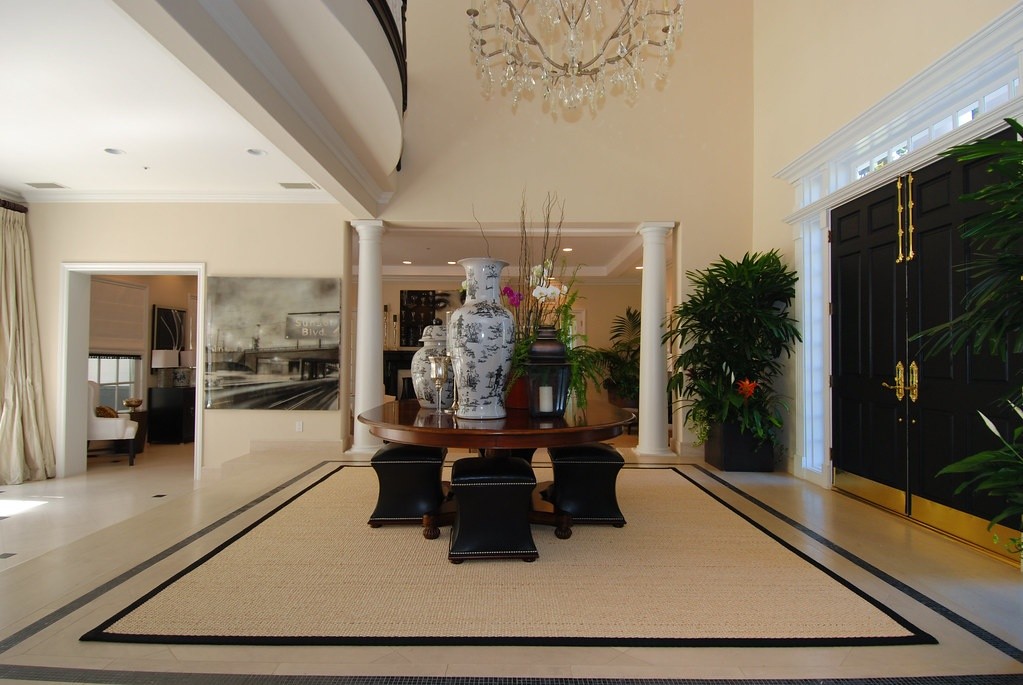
[400, 289, 435, 347]
[148, 386, 196, 445]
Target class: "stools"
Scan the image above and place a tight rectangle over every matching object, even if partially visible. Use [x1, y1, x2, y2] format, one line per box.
[367, 442, 447, 528]
[448, 457, 540, 564]
[547, 446, 627, 528]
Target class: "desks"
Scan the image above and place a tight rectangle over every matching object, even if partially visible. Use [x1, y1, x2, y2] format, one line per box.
[114, 411, 149, 453]
[358, 399, 637, 539]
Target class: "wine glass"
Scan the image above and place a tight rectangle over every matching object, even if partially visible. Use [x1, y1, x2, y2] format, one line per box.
[428, 356, 450, 416]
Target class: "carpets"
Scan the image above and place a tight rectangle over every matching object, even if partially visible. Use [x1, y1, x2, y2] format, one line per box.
[78, 466, 939, 645]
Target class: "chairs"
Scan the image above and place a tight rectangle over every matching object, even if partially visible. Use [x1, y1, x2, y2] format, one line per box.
[87, 381, 138, 467]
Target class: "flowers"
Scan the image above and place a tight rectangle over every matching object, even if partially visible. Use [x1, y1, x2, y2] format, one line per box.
[472, 188, 616, 410]
[659, 249, 802, 455]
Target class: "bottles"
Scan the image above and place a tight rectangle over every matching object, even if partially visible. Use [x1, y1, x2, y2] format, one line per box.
[448, 256, 514, 419]
[411, 318, 453, 408]
[523, 325, 570, 421]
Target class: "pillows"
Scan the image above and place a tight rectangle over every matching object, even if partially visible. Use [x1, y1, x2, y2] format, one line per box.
[96, 405, 118, 418]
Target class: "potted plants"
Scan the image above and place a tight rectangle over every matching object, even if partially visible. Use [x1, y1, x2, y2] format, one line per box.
[598, 305, 641, 408]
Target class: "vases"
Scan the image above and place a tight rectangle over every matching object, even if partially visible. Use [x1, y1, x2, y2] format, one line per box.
[505, 376, 528, 410]
[449, 258, 515, 419]
[704, 419, 775, 473]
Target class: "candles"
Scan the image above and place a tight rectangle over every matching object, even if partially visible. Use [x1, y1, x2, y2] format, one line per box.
[539, 384, 554, 413]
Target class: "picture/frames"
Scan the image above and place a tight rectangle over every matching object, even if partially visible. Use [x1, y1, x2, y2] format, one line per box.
[151, 304, 188, 374]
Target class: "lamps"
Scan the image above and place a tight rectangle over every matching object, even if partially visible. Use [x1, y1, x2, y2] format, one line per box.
[467, 0, 685, 114]
